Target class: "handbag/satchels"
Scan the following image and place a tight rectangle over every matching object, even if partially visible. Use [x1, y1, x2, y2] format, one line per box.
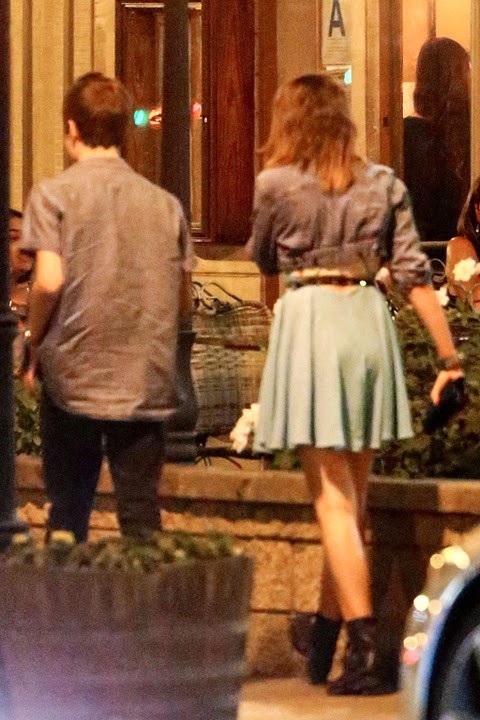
[188, 283, 272, 437]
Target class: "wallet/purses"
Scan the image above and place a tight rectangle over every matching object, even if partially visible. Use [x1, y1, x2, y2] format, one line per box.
[418, 376, 469, 434]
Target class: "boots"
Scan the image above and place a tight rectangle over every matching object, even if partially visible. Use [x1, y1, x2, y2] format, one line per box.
[326, 617, 384, 692]
[287, 613, 342, 682]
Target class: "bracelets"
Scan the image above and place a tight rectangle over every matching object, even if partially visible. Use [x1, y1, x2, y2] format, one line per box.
[437, 355, 462, 370]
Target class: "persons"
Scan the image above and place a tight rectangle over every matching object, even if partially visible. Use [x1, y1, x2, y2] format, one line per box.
[19, 70, 198, 545]
[10, 208, 34, 299]
[245, 76, 462, 696]
[401, 36, 472, 273]
